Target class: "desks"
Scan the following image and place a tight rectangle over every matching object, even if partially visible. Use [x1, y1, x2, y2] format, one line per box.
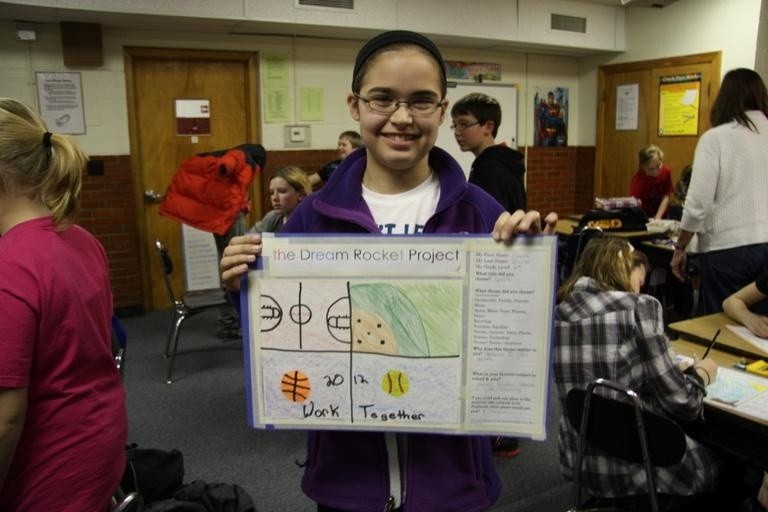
[517, 198, 766, 510]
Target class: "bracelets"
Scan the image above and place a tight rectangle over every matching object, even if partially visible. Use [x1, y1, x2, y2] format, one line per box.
[691, 364, 714, 387]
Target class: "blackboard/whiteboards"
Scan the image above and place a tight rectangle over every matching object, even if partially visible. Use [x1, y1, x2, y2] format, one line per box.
[434, 81, 520, 183]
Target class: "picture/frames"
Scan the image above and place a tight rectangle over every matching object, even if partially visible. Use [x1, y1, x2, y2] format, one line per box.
[33, 71, 87, 135]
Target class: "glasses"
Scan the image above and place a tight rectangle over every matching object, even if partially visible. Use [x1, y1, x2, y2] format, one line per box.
[352, 92, 444, 116]
[449, 119, 480, 130]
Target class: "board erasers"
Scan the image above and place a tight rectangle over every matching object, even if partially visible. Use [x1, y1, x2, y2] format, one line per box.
[447, 82, 456, 87]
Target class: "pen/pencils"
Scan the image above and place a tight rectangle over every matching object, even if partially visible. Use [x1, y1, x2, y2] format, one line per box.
[702, 329, 721, 360]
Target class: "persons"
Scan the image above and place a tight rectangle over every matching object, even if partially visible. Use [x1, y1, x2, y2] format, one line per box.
[627, 68, 767, 339]
[217, 29, 560, 512]
[161, 131, 364, 339]
[540, 90, 565, 144]
[0, 97, 126, 512]
[553, 231, 722, 508]
[450, 93, 528, 459]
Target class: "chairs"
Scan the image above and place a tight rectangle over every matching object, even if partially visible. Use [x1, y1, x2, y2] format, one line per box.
[110, 315, 128, 378]
[106, 459, 144, 511]
[152, 240, 244, 385]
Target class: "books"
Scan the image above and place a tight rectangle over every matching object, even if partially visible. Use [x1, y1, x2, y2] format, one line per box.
[591, 192, 641, 214]
